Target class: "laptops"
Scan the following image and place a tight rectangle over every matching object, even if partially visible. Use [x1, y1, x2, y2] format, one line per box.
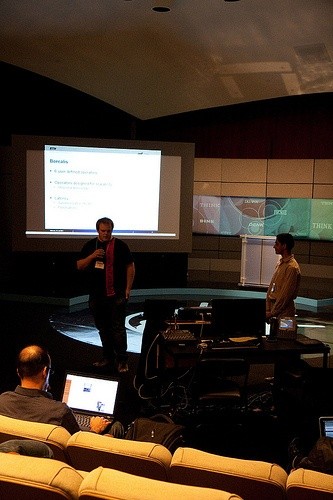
[60, 370, 121, 431]
[319, 416, 333, 440]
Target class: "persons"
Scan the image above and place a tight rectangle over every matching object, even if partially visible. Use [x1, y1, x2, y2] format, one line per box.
[0, 344, 124, 439]
[76, 217, 136, 372]
[265, 233, 302, 337]
[290, 431, 333, 476]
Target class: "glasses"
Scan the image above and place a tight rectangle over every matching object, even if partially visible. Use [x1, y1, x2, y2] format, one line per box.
[48, 368, 54, 374]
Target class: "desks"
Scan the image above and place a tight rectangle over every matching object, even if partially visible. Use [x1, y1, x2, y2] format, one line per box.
[161, 333, 328, 388]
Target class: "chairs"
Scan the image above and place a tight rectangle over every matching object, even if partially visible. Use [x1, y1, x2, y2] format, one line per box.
[197, 357, 250, 407]
[0, 414, 332, 500]
